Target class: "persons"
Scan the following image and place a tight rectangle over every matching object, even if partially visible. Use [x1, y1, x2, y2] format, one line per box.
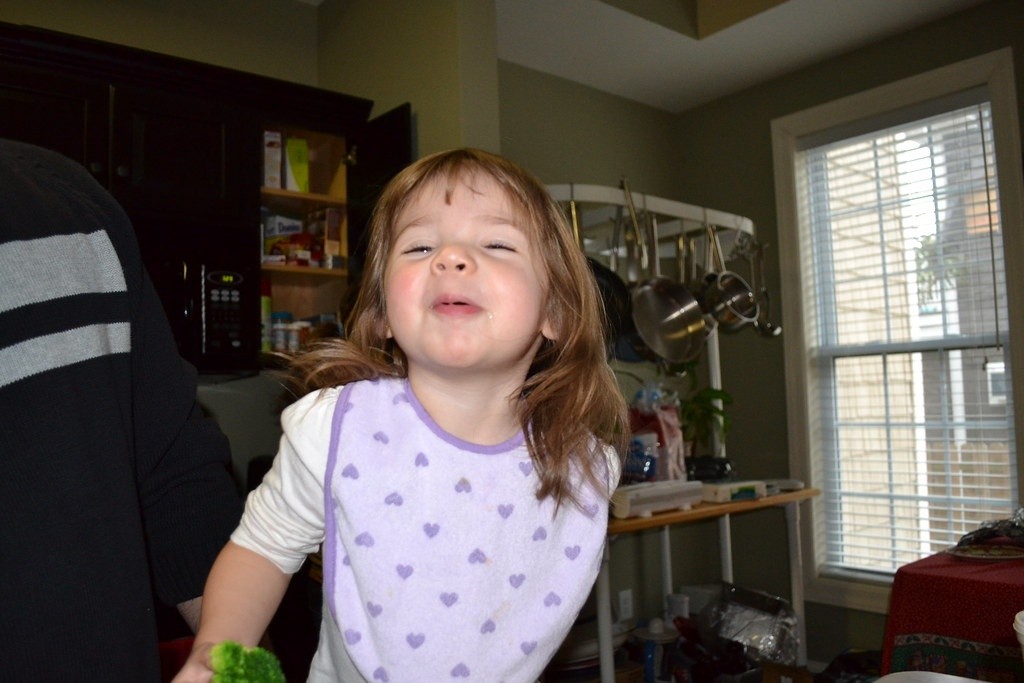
[0, 138, 292, 683]
[171, 147, 632, 683]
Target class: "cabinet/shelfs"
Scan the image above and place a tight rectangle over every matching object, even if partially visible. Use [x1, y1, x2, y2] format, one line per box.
[0, 21, 411, 372]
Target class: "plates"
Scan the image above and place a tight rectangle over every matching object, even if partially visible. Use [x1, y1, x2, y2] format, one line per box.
[946, 547, 1024, 559]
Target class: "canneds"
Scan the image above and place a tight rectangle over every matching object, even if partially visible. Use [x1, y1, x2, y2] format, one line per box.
[270, 311, 311, 354]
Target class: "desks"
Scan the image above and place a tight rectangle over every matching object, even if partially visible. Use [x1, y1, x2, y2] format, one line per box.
[593, 486, 823, 683]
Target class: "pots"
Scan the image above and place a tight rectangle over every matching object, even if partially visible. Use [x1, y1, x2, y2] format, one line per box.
[622, 183, 707, 363]
[702, 221, 760, 331]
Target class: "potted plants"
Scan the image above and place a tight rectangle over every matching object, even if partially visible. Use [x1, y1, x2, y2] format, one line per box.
[670, 385, 739, 484]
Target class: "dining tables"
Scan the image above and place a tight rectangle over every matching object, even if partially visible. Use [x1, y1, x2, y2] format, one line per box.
[880, 550, 1024, 683]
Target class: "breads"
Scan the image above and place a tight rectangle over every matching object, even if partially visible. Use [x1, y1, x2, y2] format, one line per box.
[953, 544, 1024, 557]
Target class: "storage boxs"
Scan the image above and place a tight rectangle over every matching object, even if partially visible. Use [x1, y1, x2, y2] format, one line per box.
[262, 130, 310, 192]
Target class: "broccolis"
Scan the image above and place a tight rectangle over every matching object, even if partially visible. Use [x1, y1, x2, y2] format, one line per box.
[208, 641, 287, 683]
